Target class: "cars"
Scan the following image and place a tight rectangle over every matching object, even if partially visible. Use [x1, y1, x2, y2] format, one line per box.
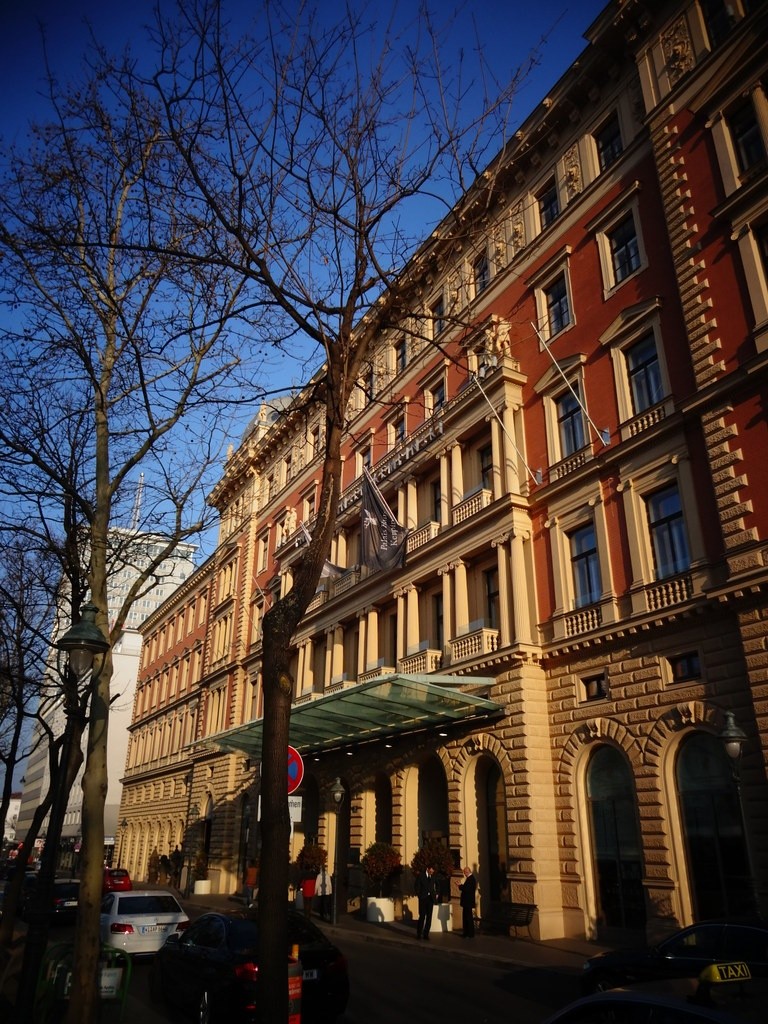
[99, 890, 191, 963]
[579, 919, 768, 1000]
[0, 843, 40, 882]
[149, 905, 349, 1024]
[16, 869, 81, 926]
[101, 867, 133, 896]
[542, 960, 768, 1024]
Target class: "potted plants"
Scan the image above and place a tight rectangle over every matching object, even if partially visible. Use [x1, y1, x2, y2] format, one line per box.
[192, 853, 211, 895]
[360, 842, 404, 924]
[410, 839, 453, 934]
[294, 842, 328, 910]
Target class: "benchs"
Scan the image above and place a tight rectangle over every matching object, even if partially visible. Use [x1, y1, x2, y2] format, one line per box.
[472, 900, 538, 945]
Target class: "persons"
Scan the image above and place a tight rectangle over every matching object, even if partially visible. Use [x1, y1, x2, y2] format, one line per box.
[414, 865, 438, 940]
[243, 859, 257, 909]
[455, 868, 476, 940]
[299, 865, 333, 920]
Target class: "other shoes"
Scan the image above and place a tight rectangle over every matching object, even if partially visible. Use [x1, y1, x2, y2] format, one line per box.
[416, 935, 421, 940]
[423, 935, 430, 940]
